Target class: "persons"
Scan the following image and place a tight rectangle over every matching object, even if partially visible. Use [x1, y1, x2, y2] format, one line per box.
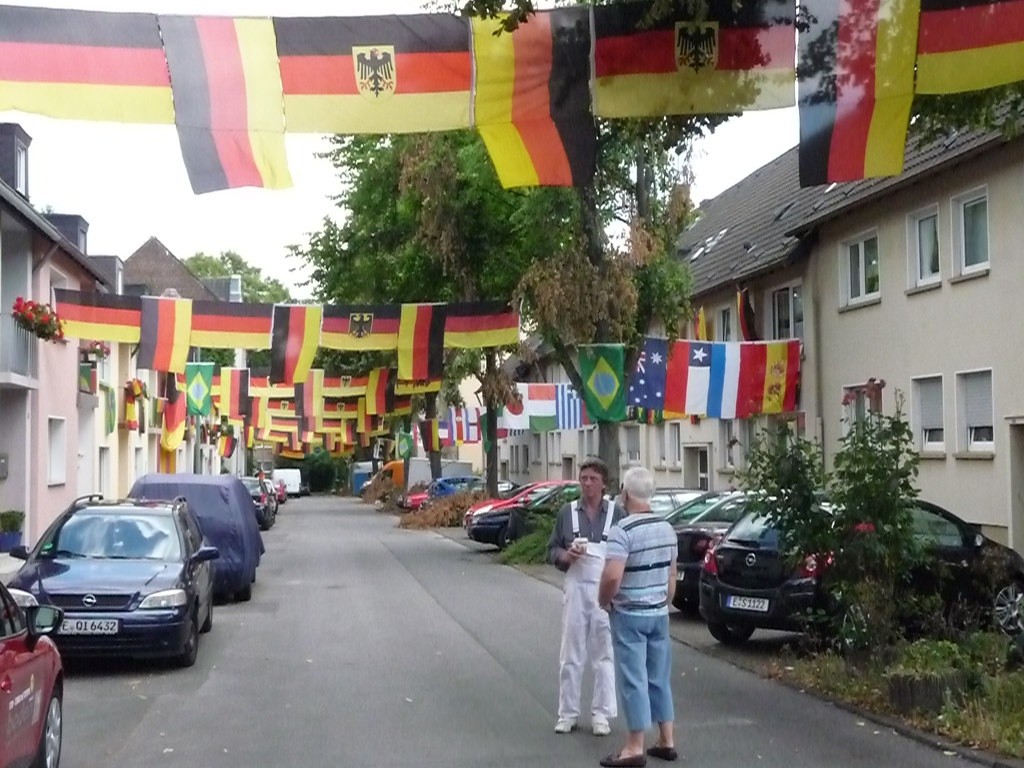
[546, 458, 628, 737]
[598, 468, 678, 768]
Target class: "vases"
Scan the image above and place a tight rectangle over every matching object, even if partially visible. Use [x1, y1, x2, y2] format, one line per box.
[87, 353, 101, 362]
[17, 317, 52, 342]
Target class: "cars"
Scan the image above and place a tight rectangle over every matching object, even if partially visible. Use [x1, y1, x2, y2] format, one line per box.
[238, 469, 311, 530]
[463, 480, 581, 552]
[613, 488, 842, 611]
[698, 499, 1023, 658]
[1, 582, 63, 768]
[396, 476, 520, 511]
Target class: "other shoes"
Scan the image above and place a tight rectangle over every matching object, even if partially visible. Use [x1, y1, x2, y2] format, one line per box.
[600, 753, 646, 768]
[592, 715, 610, 736]
[555, 716, 577, 733]
[646, 744, 676, 761]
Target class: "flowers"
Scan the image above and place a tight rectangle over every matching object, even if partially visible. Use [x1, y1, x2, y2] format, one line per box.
[126, 378, 150, 400]
[214, 424, 227, 438]
[11, 297, 67, 345]
[86, 340, 111, 360]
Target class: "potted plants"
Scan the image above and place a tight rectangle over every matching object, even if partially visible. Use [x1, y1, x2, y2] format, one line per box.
[881, 638, 977, 719]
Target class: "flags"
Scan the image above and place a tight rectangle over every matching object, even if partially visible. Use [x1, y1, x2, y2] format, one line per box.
[51, 288, 525, 385]
[155, 366, 443, 459]
[577, 338, 800, 425]
[374, 380, 612, 464]
[0, 0, 1023, 193]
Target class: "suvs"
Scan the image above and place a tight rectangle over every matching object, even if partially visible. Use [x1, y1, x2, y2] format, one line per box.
[5, 493, 221, 667]
[126, 472, 265, 602]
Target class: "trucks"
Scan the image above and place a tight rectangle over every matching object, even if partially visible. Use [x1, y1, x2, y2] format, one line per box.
[359, 460, 472, 504]
